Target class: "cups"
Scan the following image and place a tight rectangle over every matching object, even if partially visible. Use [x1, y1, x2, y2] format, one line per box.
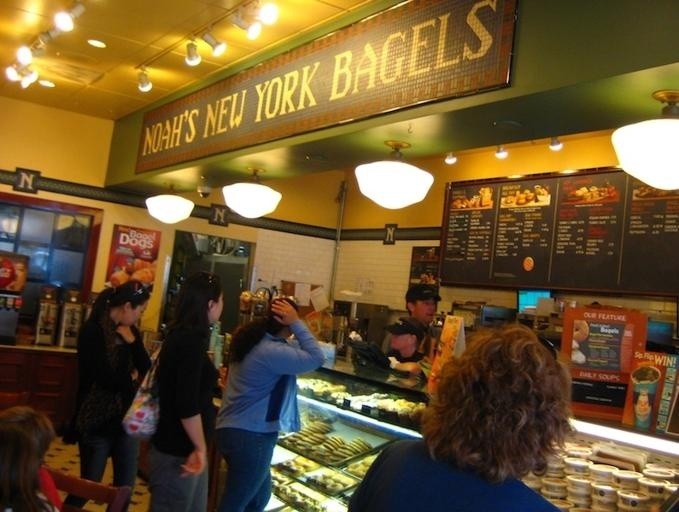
[631, 367, 661, 431]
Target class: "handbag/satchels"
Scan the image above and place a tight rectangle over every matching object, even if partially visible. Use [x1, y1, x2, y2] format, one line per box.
[122, 388, 160, 440]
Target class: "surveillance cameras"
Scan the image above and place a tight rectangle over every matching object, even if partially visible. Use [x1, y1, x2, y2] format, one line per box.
[197, 185, 212, 198]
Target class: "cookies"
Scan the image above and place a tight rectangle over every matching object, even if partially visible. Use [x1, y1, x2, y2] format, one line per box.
[271, 420, 388, 512]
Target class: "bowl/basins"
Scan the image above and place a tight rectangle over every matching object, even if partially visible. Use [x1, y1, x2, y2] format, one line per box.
[519, 442, 678, 511]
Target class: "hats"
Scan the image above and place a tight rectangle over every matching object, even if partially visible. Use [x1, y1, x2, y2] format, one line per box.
[406, 283, 442, 302]
[383, 317, 424, 338]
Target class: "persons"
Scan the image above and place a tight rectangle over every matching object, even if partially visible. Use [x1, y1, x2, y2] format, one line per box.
[0, 405, 63, 512]
[0, 422, 60, 512]
[214, 296, 325, 512]
[384, 316, 426, 376]
[346, 323, 573, 512]
[381, 282, 442, 354]
[144, 270, 224, 512]
[61, 279, 153, 512]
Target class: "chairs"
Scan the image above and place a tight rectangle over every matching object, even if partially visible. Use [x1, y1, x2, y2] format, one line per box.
[43, 464, 131, 512]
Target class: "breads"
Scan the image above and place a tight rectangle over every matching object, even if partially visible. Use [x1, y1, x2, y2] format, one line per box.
[295, 377, 428, 418]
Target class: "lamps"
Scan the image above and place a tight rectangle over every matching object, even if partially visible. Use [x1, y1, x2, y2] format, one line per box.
[135, 0, 277, 93]
[354, 140, 434, 209]
[611, 90, 679, 191]
[146, 182, 194, 224]
[7, 4, 86, 90]
[222, 168, 282, 218]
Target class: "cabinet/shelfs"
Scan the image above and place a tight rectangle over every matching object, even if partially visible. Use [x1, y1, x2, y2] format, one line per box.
[0, 342, 77, 434]
[265, 394, 425, 512]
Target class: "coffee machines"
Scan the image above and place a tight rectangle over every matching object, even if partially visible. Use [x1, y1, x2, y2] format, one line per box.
[35, 287, 103, 347]
[330, 298, 389, 362]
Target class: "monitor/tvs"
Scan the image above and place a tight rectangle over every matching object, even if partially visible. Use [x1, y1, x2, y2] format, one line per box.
[517, 288, 552, 315]
[349, 340, 391, 368]
